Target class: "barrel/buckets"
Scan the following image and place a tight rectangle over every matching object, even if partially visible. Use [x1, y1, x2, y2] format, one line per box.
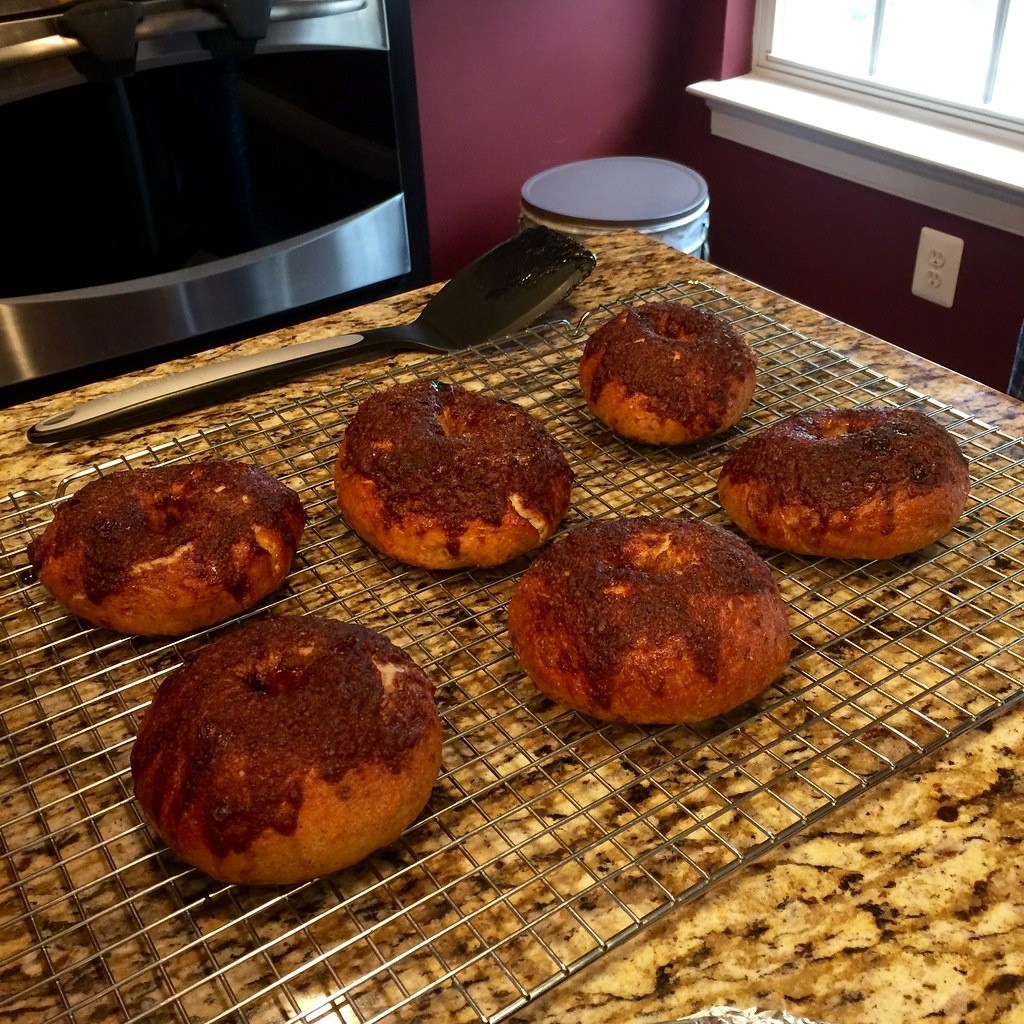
[517, 156, 710, 265]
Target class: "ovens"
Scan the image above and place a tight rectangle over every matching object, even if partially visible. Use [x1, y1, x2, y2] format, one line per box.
[0, 0, 409, 400]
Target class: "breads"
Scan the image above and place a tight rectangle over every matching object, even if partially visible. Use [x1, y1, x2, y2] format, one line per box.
[509, 515, 790, 725]
[716, 406, 970, 558]
[26, 458, 306, 636]
[333, 381, 573, 569]
[579, 300, 758, 447]
[130, 616, 442, 886]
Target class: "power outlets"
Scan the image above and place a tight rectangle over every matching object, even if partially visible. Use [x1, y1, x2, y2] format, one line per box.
[912, 227, 964, 307]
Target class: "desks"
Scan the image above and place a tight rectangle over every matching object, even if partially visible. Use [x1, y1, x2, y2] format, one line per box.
[0, 225, 1024, 1024]
